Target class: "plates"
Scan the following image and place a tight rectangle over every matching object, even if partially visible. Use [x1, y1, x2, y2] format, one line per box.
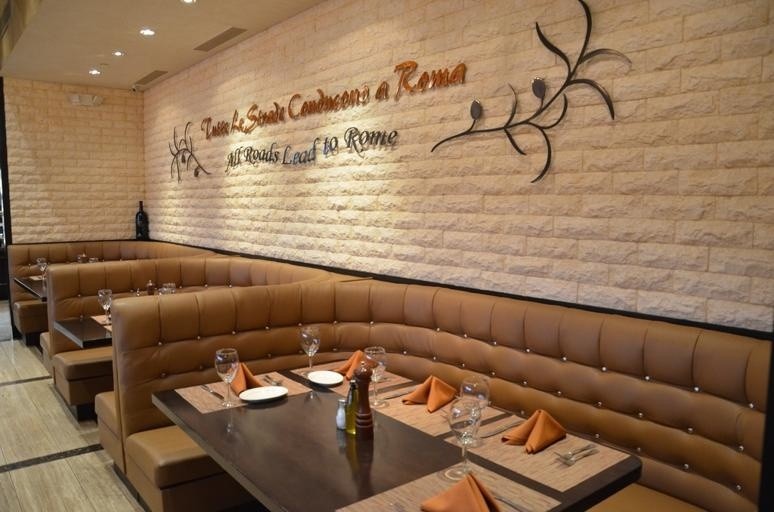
[239, 386, 288, 404]
[307, 371, 345, 387]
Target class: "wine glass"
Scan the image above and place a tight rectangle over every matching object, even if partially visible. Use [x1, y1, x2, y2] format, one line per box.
[215, 348, 240, 407]
[365, 347, 389, 408]
[37, 257, 47, 281]
[458, 377, 489, 430]
[98, 288, 113, 324]
[302, 327, 322, 374]
[447, 398, 482, 476]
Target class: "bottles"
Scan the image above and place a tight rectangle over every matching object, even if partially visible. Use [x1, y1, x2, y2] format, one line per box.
[346, 381, 358, 435]
[335, 399, 347, 430]
[135, 200, 149, 241]
[355, 363, 374, 441]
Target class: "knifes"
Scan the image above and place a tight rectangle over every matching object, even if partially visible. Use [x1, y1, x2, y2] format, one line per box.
[482, 420, 525, 439]
[201, 385, 224, 402]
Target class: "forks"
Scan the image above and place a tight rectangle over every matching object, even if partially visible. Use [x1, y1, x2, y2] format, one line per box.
[557, 449, 600, 467]
[553, 444, 595, 460]
